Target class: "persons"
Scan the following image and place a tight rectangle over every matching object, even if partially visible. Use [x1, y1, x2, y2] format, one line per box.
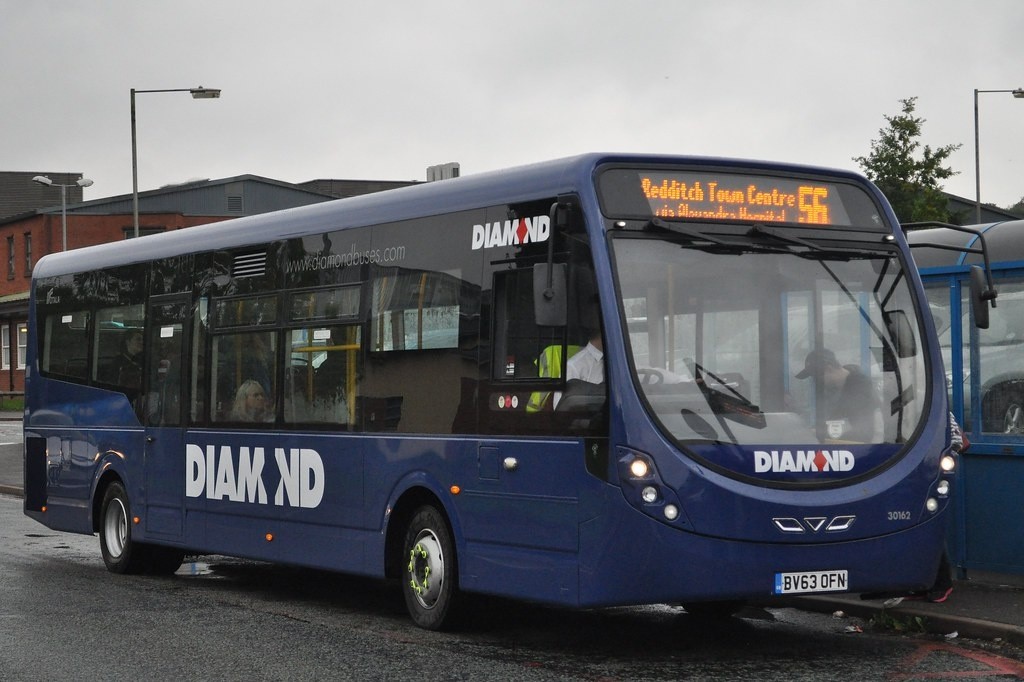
[552, 303, 693, 410]
[904, 409, 963, 602]
[110, 329, 350, 425]
[795, 348, 884, 444]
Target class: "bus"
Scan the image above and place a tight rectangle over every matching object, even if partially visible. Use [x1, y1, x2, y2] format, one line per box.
[23, 151, 1000, 632]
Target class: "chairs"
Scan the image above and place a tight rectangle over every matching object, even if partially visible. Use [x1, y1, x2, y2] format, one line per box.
[286, 365, 314, 398]
[538, 346, 581, 406]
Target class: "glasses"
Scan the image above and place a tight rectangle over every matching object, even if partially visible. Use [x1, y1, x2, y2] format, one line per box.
[246, 391, 265, 398]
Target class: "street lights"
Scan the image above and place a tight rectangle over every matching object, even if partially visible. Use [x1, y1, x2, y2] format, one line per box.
[32, 174, 94, 253]
[130, 85, 222, 237]
[973, 88, 1024, 224]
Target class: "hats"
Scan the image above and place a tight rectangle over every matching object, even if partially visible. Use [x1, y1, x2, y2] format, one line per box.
[157, 359, 170, 373]
[795, 348, 835, 379]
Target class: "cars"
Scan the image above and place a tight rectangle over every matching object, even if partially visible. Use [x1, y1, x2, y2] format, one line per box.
[933, 291, 1024, 435]
[637, 295, 972, 416]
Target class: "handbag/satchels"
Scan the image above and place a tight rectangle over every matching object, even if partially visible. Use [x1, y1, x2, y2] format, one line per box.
[952, 422, 970, 455]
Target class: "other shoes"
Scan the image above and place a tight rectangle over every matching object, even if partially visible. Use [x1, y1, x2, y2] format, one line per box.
[929, 579, 954, 603]
